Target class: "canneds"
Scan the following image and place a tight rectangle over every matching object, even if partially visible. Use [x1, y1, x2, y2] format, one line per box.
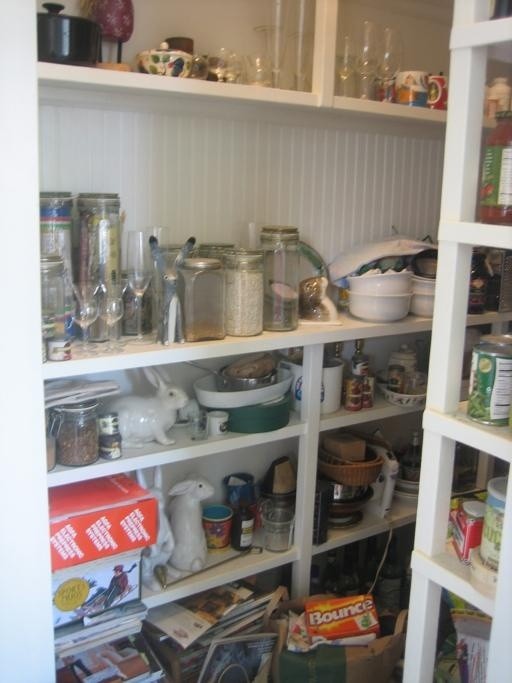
[467, 333, 510, 426]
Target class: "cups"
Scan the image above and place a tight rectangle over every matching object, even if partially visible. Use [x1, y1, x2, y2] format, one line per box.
[215, 47, 239, 84]
[263, 508, 294, 552]
[429, 74, 446, 108]
[221, 472, 255, 505]
[243, 53, 271, 87]
[201, 505, 232, 550]
[250, 1, 312, 93]
[186, 405, 229, 439]
[394, 69, 442, 107]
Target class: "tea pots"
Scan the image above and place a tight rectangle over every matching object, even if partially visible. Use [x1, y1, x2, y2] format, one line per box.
[384, 343, 418, 390]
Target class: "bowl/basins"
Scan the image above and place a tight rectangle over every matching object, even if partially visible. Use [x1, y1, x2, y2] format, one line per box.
[189, 369, 294, 410]
[346, 267, 436, 322]
[376, 382, 426, 407]
[137, 52, 193, 77]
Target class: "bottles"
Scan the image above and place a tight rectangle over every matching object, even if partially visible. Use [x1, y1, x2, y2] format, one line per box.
[58, 398, 98, 466]
[403, 429, 421, 481]
[154, 224, 300, 342]
[469, 248, 488, 315]
[281, 537, 413, 612]
[471, 109, 510, 225]
[487, 75, 512, 112]
[271, 339, 370, 377]
[38, 190, 123, 360]
[234, 504, 255, 550]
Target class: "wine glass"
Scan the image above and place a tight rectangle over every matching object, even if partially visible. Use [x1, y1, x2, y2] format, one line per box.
[335, 31, 355, 98]
[376, 25, 398, 101]
[354, 21, 377, 99]
[128, 231, 154, 348]
[73, 264, 123, 357]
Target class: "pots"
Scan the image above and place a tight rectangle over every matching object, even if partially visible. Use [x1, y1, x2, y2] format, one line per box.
[183, 359, 277, 390]
[319, 478, 373, 529]
[37, 1, 104, 66]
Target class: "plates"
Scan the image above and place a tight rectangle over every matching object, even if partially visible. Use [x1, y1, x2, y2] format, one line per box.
[389, 479, 419, 506]
